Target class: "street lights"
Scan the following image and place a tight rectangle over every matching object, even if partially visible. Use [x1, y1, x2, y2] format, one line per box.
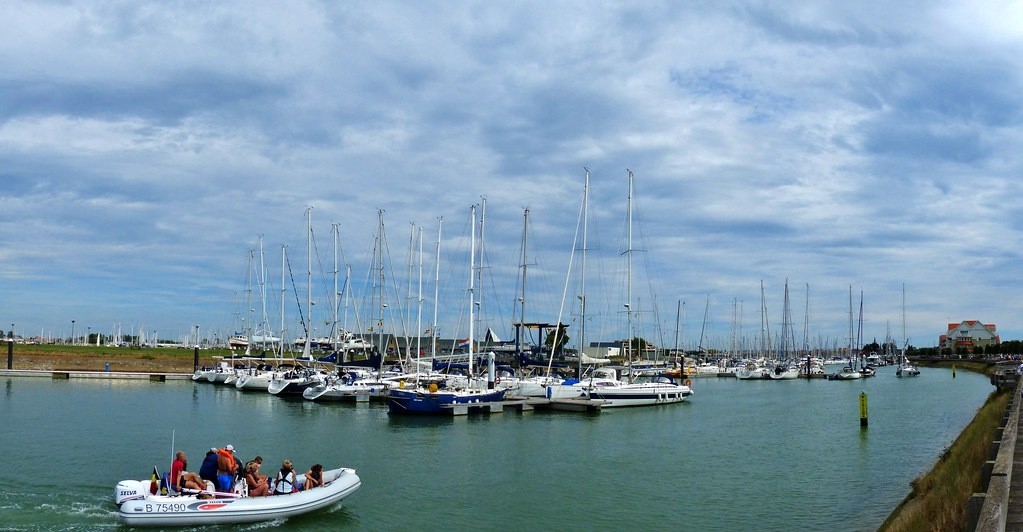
[72, 320, 75, 345]
[195, 326, 200, 346]
[11, 324, 14, 342]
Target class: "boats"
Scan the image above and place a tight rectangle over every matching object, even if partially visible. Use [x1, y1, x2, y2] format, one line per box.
[114, 428, 362, 527]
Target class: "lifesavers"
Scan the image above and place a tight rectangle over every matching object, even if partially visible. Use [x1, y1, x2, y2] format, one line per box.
[686, 380, 691, 387]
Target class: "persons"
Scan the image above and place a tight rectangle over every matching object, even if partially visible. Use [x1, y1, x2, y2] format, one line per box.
[172, 445, 324, 497]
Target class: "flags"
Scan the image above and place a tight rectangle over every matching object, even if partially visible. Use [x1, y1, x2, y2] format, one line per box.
[149, 466, 159, 495]
[161, 478, 170, 496]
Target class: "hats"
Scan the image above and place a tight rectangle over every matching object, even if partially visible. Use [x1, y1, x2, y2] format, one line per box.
[225, 445, 236, 452]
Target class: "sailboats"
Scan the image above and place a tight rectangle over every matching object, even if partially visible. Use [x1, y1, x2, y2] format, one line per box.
[189, 166, 921, 418]
